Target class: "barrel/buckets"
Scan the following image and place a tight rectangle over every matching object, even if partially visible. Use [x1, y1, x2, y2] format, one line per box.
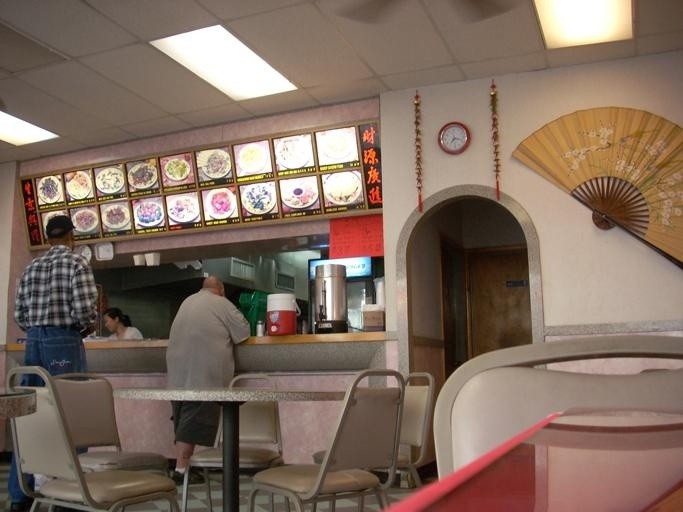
[266, 292, 301, 336]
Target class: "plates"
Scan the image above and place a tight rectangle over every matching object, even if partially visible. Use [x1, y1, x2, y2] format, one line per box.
[323, 171, 362, 203]
[201, 149, 231, 180]
[241, 183, 277, 214]
[165, 159, 190, 181]
[237, 145, 267, 173]
[280, 180, 318, 209]
[205, 189, 235, 219]
[134, 202, 163, 227]
[102, 205, 129, 228]
[67, 171, 91, 199]
[42, 211, 65, 241]
[71, 209, 98, 234]
[275, 139, 310, 169]
[36, 175, 62, 205]
[95, 169, 124, 194]
[127, 163, 158, 190]
[166, 196, 199, 222]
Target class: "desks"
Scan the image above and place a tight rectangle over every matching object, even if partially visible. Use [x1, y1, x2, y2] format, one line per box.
[113, 386, 349, 512]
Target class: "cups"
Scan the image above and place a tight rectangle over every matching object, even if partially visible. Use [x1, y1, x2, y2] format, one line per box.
[376, 281, 385, 305]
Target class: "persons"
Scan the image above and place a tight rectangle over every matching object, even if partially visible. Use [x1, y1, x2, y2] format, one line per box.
[8, 214, 100, 511]
[102, 308, 143, 341]
[162, 273, 253, 485]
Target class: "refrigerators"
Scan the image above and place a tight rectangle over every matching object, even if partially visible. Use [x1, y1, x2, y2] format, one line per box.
[307, 255, 377, 331]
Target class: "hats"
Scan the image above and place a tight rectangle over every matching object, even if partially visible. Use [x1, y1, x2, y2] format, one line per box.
[45, 216, 75, 237]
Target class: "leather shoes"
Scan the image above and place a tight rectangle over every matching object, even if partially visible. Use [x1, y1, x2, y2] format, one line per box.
[10, 501, 31, 511]
[172, 467, 204, 485]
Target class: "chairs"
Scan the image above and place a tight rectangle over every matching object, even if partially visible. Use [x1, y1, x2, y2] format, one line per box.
[178, 374, 290, 512]
[310, 373, 435, 512]
[247, 368, 405, 512]
[6, 366, 181, 512]
[427, 330, 682, 484]
[43, 372, 174, 512]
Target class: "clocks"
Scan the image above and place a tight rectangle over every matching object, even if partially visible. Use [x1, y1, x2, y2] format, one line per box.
[435, 121, 469, 155]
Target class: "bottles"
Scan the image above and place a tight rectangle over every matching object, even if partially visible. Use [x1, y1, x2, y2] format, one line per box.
[256, 321, 265, 336]
